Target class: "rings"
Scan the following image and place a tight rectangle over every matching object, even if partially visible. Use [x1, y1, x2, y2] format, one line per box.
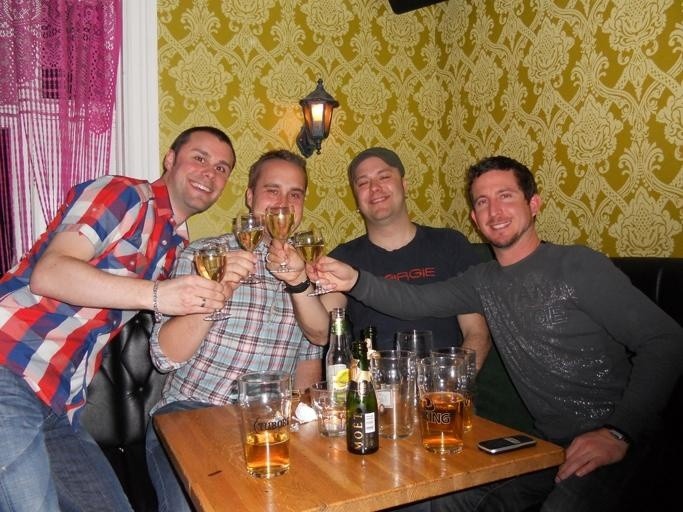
[200, 297, 206, 307]
[265, 253, 271, 263]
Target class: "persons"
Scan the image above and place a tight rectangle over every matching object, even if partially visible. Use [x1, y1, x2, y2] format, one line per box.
[264, 147, 493, 381]
[302, 156, 683, 511]
[0, 125, 238, 512]
[142, 148, 350, 512]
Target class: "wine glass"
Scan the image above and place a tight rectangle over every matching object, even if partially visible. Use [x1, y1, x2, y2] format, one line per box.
[235, 204, 337, 297]
[193, 244, 233, 321]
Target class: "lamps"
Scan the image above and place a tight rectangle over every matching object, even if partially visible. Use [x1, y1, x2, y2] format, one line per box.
[295, 78, 340, 158]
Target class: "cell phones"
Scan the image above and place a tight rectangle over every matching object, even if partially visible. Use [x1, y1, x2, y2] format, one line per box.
[478, 434, 537, 454]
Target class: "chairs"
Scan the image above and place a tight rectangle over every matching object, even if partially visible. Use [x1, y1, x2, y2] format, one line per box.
[76, 307, 159, 511]
[609, 256, 682, 324]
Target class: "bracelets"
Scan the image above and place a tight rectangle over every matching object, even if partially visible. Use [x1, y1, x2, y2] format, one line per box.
[601, 424, 635, 447]
[284, 275, 310, 294]
[150, 280, 164, 324]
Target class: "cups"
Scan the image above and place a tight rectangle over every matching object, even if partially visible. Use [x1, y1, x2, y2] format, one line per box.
[237, 370, 292, 479]
[309, 381, 349, 436]
[371, 327, 477, 455]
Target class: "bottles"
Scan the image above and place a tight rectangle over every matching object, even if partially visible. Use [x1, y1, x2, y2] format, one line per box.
[326, 308, 381, 455]
